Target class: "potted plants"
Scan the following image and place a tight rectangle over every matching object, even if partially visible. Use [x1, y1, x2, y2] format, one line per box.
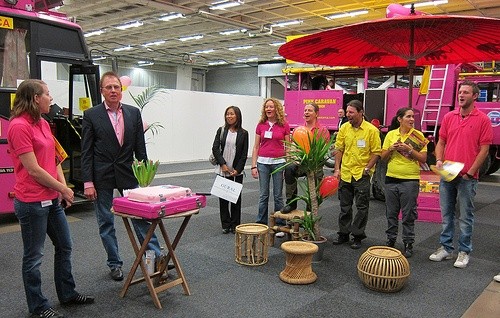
[269, 126, 339, 263]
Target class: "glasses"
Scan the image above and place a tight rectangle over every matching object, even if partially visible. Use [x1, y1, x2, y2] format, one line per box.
[101, 84, 121, 90]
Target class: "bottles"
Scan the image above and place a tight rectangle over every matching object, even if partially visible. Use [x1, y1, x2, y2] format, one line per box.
[145, 249, 156, 274]
[157, 246, 169, 279]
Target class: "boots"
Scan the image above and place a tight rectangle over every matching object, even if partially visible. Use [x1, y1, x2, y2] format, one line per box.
[280, 181, 297, 213]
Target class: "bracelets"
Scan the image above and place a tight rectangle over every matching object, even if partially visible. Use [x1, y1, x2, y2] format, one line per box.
[365, 168, 370, 173]
[435, 160, 443, 163]
[251, 167, 257, 170]
[408, 149, 414, 155]
[334, 170, 339, 172]
[465, 172, 474, 179]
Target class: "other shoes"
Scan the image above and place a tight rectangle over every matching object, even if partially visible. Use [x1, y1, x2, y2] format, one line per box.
[60, 294, 95, 306]
[402, 241, 413, 257]
[351, 234, 361, 248]
[222, 227, 231, 233]
[332, 231, 349, 245]
[32, 306, 64, 318]
[381, 238, 397, 249]
[275, 231, 286, 238]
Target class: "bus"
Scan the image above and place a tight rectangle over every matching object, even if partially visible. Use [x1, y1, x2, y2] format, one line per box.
[282, 34, 500, 179]
[0, 0, 118, 216]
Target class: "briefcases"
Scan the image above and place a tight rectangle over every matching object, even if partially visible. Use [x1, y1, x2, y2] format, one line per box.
[112, 194, 207, 219]
[127, 184, 193, 203]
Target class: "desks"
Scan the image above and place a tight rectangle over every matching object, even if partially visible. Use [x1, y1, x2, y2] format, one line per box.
[111, 206, 199, 308]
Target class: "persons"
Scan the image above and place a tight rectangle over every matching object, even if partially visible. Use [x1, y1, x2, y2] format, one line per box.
[428, 80, 493, 268]
[212, 106, 249, 233]
[333, 100, 381, 248]
[281, 102, 330, 231]
[326, 80, 334, 90]
[312, 75, 328, 90]
[382, 107, 428, 257]
[7, 79, 95, 318]
[251, 99, 290, 237]
[80, 72, 175, 281]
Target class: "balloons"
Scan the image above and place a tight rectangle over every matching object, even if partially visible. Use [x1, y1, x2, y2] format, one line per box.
[319, 176, 339, 198]
[293, 126, 314, 154]
[119, 76, 131, 86]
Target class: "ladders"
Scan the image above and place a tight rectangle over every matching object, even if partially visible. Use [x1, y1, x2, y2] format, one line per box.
[421, 64, 448, 142]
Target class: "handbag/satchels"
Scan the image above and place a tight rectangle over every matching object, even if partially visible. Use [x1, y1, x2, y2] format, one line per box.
[209, 126, 225, 166]
[210, 171, 244, 204]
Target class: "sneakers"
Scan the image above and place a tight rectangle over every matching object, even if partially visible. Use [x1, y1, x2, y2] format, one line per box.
[429, 246, 454, 262]
[453, 251, 471, 268]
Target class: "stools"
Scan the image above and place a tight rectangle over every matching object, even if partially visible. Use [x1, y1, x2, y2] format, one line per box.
[357, 246, 410, 293]
[234, 223, 269, 266]
[270, 209, 311, 247]
[279, 240, 319, 285]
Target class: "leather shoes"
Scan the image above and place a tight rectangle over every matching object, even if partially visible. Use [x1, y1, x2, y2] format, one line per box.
[111, 268, 123, 281]
[154, 262, 175, 270]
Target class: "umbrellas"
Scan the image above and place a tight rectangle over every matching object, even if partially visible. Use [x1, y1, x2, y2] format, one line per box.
[278, 2, 500, 105]
